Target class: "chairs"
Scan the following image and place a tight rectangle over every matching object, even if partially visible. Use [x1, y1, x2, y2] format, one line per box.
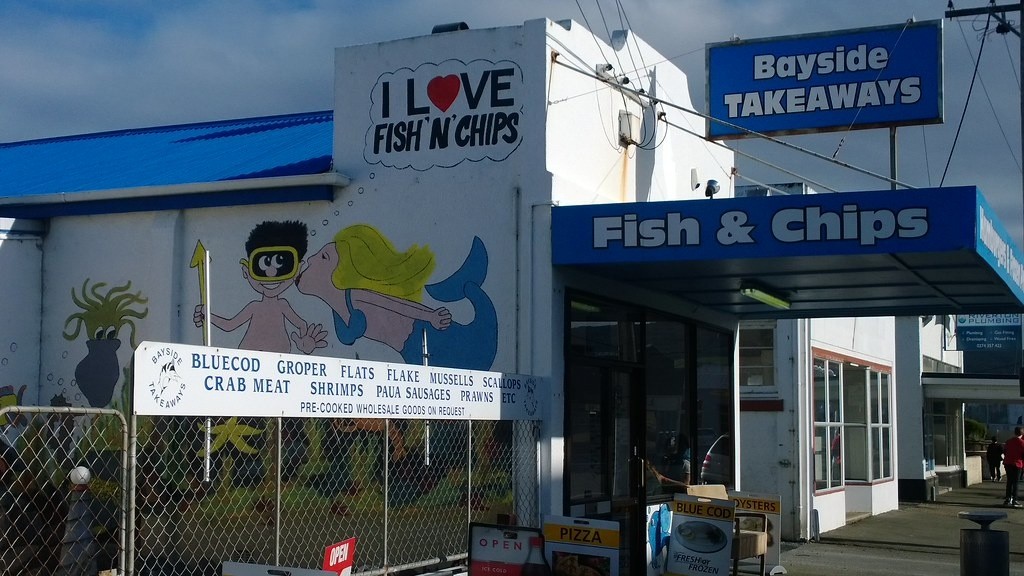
[685, 483, 768, 576]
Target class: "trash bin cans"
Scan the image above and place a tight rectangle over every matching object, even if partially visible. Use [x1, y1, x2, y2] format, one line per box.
[958, 511, 1010, 576]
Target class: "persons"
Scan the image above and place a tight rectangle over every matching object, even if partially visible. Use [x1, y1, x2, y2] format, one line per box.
[648, 433, 690, 492]
[831, 428, 841, 480]
[1003, 427, 1024, 507]
[986, 436, 1003, 482]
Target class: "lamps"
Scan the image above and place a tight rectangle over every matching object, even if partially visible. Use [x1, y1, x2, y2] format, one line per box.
[739, 286, 790, 312]
[705, 179, 720, 198]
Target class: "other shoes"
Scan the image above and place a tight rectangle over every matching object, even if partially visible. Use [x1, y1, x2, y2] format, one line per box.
[1003, 501, 1013, 508]
[1013, 500, 1023, 508]
[992, 477, 996, 481]
[997, 476, 1003, 481]
